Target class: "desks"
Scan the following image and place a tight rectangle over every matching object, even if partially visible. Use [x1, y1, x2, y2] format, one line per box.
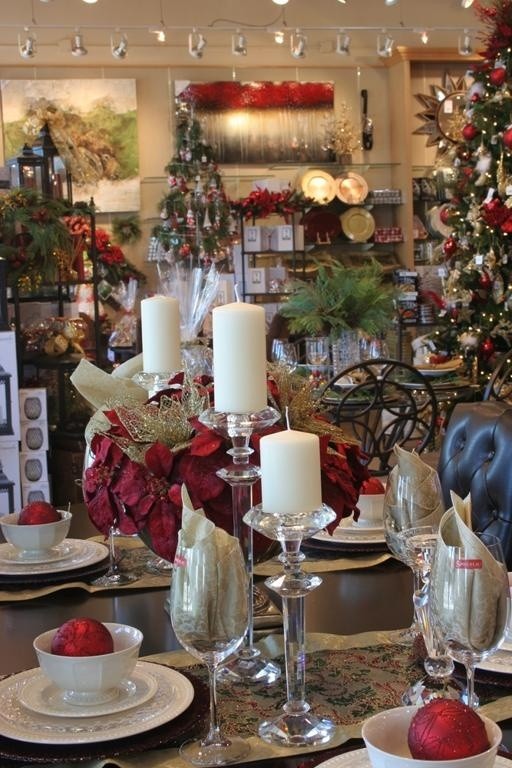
[315, 372, 479, 450]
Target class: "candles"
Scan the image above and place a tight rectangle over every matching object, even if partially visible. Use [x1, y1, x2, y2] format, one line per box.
[261, 405, 323, 512]
[211, 279, 269, 413]
[142, 293, 183, 377]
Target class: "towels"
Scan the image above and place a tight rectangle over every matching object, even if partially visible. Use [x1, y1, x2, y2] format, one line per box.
[385, 438, 440, 541]
[170, 484, 246, 651]
[427, 494, 507, 647]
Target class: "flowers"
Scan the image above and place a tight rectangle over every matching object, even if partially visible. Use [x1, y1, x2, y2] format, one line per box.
[230, 185, 322, 223]
[76, 349, 381, 575]
[81, 224, 141, 346]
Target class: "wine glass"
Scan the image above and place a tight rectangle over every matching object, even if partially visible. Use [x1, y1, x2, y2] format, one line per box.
[169, 537, 251, 767]
[384, 455, 510, 713]
[81, 448, 140, 588]
[267, 332, 391, 382]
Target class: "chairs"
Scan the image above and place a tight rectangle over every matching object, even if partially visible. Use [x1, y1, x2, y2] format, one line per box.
[319, 356, 435, 478]
[438, 397, 511, 570]
[484, 347, 511, 400]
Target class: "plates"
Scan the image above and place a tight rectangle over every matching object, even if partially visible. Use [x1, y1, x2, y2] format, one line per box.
[323, 396, 393, 403]
[23, 670, 155, 722]
[1, 661, 213, 766]
[331, 382, 362, 389]
[375, 228, 404, 243]
[314, 744, 512, 768]
[2, 542, 86, 564]
[0, 538, 110, 576]
[417, 367, 458, 377]
[371, 188, 401, 205]
[301, 169, 376, 242]
[305, 525, 388, 550]
[330, 517, 391, 535]
[395, 380, 453, 391]
[1, 661, 195, 746]
[0, 541, 117, 586]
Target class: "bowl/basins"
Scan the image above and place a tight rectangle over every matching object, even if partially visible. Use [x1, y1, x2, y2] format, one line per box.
[1, 510, 72, 562]
[32, 622, 144, 706]
[361, 701, 502, 766]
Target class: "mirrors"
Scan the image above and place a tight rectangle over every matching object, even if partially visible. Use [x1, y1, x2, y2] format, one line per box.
[410, 69, 477, 169]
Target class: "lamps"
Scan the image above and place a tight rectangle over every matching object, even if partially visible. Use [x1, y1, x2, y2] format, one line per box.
[31, 114, 75, 207]
[6, 142, 51, 197]
[7, 20, 476, 62]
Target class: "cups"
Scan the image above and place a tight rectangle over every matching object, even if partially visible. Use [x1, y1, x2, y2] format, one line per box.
[419, 302, 434, 325]
[348, 477, 392, 526]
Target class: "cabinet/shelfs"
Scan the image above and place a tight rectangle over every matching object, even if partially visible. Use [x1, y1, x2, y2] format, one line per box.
[4, 194, 103, 448]
[231, 188, 307, 307]
[280, 164, 455, 324]
